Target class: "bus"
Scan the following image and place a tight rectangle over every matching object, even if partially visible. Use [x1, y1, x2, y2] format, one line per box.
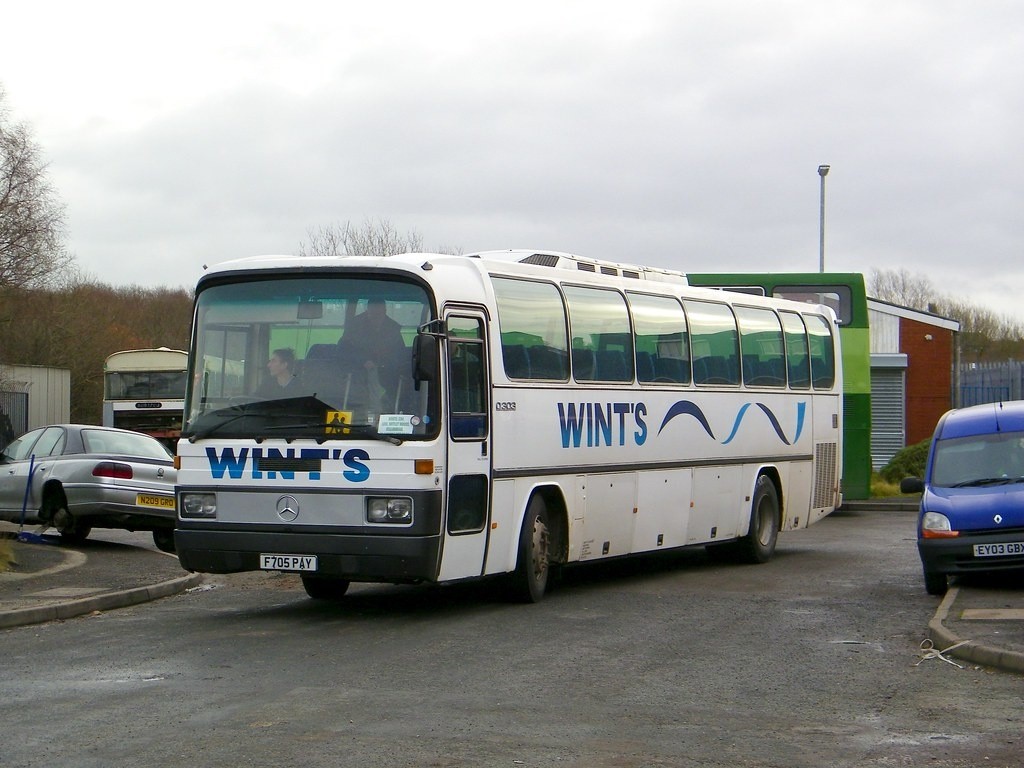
[268, 270, 873, 503]
[174, 245, 845, 604]
[102, 345, 244, 452]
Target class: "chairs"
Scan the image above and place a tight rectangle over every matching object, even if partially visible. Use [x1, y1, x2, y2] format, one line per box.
[127, 382, 150, 398]
[306, 344, 337, 361]
[502, 344, 831, 385]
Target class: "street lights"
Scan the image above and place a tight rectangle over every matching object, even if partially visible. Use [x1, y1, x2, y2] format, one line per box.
[817, 164, 830, 273]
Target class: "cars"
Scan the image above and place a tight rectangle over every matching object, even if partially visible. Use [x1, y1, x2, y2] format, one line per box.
[0, 424, 177, 551]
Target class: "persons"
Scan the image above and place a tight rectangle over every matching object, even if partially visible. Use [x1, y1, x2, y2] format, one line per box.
[336, 296, 407, 414]
[448, 330, 482, 412]
[249, 350, 307, 402]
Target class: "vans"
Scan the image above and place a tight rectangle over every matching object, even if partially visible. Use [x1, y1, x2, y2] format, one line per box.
[918, 400, 1024, 594]
